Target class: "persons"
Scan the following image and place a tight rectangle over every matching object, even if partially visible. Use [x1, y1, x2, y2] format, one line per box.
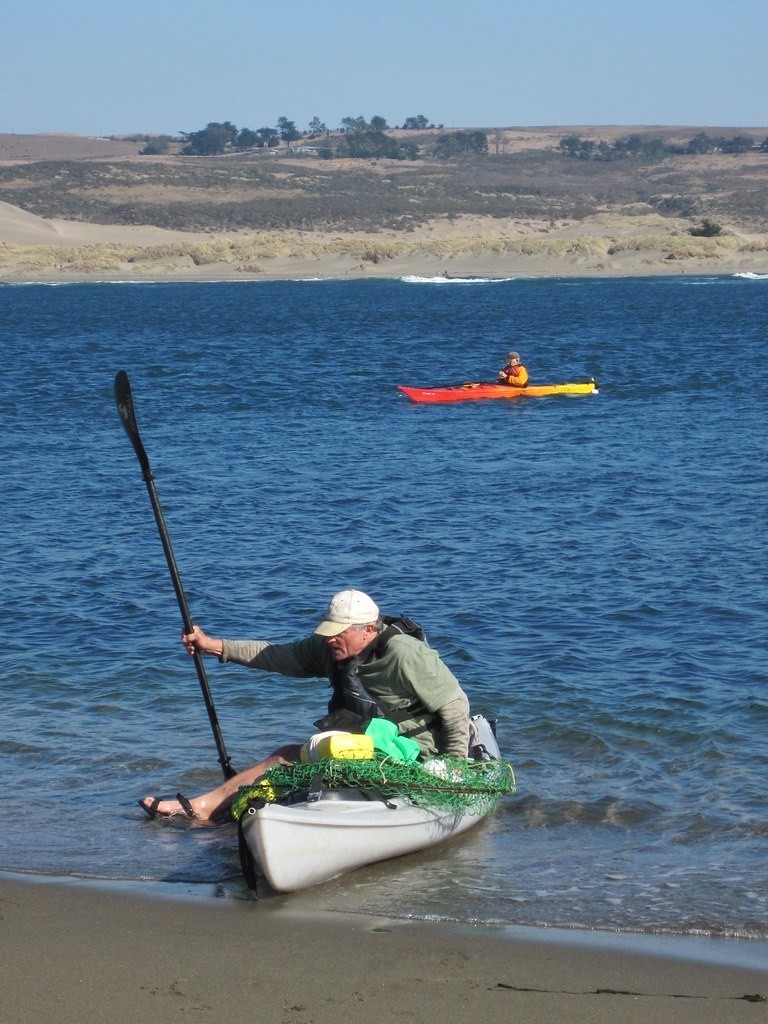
[139, 590, 470, 820]
[497, 352, 528, 388]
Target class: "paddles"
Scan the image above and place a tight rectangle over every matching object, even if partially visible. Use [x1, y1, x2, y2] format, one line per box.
[115, 368, 232, 775]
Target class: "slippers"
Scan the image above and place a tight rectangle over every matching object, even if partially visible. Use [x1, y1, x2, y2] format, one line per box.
[138, 792, 204, 829]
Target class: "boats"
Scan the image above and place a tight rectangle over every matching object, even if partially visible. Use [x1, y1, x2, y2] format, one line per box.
[396, 379, 601, 404]
[240, 712, 503, 894]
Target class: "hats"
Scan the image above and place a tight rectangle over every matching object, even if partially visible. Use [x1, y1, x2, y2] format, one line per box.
[314, 589, 379, 637]
[507, 352, 519, 359]
